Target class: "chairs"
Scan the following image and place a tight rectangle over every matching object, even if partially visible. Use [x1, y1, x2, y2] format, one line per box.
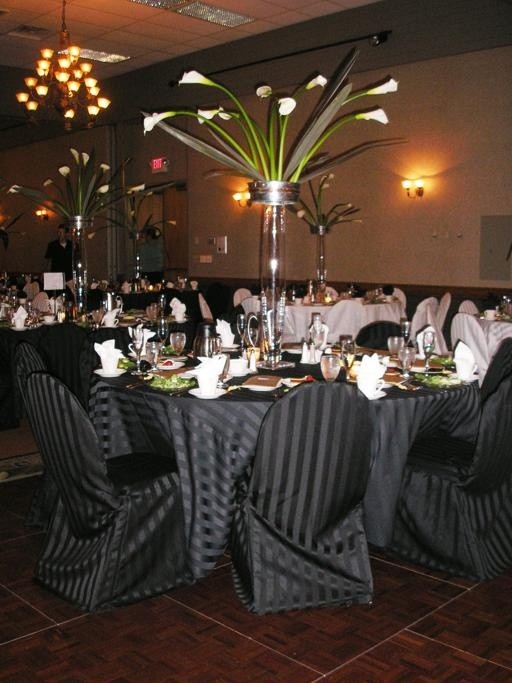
[14, 336, 66, 531]
[393, 335, 511, 581]
[25, 370, 195, 616]
[231, 383, 378, 619]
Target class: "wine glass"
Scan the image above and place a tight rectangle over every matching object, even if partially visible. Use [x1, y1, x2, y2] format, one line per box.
[146, 292, 168, 322]
[129, 323, 186, 376]
[340, 339, 357, 382]
[289, 278, 385, 305]
[237, 314, 250, 350]
[386, 315, 437, 378]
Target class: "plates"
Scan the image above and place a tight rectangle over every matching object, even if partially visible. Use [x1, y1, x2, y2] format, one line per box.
[100, 324, 120, 328]
[10, 325, 28, 331]
[281, 377, 298, 388]
[41, 321, 57, 325]
[450, 372, 479, 383]
[187, 385, 224, 399]
[280, 348, 302, 354]
[184, 369, 198, 375]
[244, 378, 283, 394]
[481, 316, 500, 321]
[365, 390, 388, 401]
[94, 367, 127, 377]
[219, 344, 240, 350]
[227, 370, 251, 378]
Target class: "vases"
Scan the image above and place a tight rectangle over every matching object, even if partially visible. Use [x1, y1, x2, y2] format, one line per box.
[312, 222, 327, 308]
[65, 215, 95, 314]
[134, 236, 143, 279]
[248, 183, 304, 370]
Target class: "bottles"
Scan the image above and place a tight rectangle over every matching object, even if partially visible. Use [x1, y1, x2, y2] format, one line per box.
[249, 348, 258, 374]
[242, 348, 248, 359]
[306, 313, 329, 351]
[309, 341, 318, 364]
[300, 341, 310, 364]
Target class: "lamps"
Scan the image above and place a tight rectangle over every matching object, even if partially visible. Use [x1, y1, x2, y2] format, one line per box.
[401, 179, 423, 200]
[16, 1, 114, 132]
[230, 190, 250, 210]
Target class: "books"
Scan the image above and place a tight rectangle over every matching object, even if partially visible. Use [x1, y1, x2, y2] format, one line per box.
[241, 375, 284, 390]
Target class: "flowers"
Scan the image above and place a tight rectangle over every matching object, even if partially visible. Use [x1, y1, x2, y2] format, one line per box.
[86, 182, 176, 258]
[141, 44, 409, 184]
[286, 173, 364, 234]
[0, 146, 146, 234]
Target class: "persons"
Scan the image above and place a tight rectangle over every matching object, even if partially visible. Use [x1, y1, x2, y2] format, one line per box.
[44, 223, 81, 281]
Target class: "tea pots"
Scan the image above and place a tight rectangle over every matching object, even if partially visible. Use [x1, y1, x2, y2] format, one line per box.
[192, 319, 222, 360]
[103, 289, 123, 316]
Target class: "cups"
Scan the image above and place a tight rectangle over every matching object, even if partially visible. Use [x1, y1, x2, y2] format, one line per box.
[98, 352, 121, 371]
[221, 334, 235, 345]
[173, 318, 188, 323]
[357, 370, 385, 395]
[172, 311, 186, 319]
[319, 353, 341, 384]
[10, 313, 26, 327]
[484, 308, 498, 318]
[229, 358, 248, 372]
[456, 359, 480, 376]
[41, 314, 56, 322]
[104, 318, 118, 324]
[193, 365, 220, 392]
[500, 294, 512, 315]
